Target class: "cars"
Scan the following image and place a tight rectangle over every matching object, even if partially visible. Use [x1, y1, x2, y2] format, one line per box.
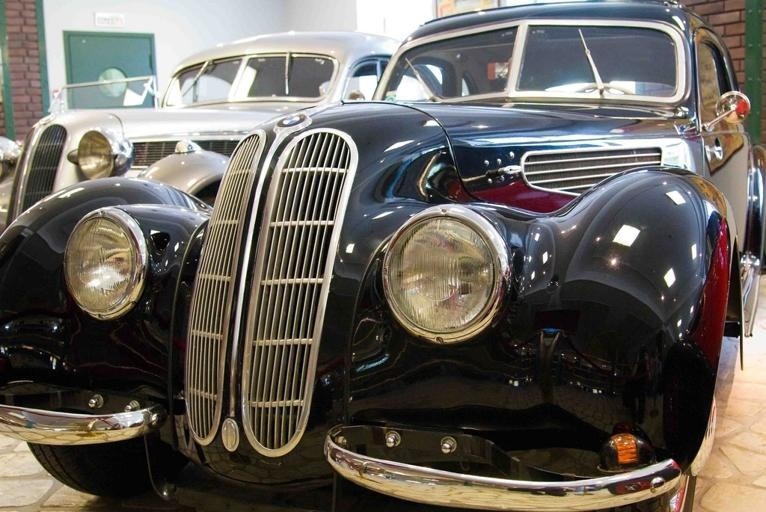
[0, 1, 766, 512]
[3, 30, 470, 219]
